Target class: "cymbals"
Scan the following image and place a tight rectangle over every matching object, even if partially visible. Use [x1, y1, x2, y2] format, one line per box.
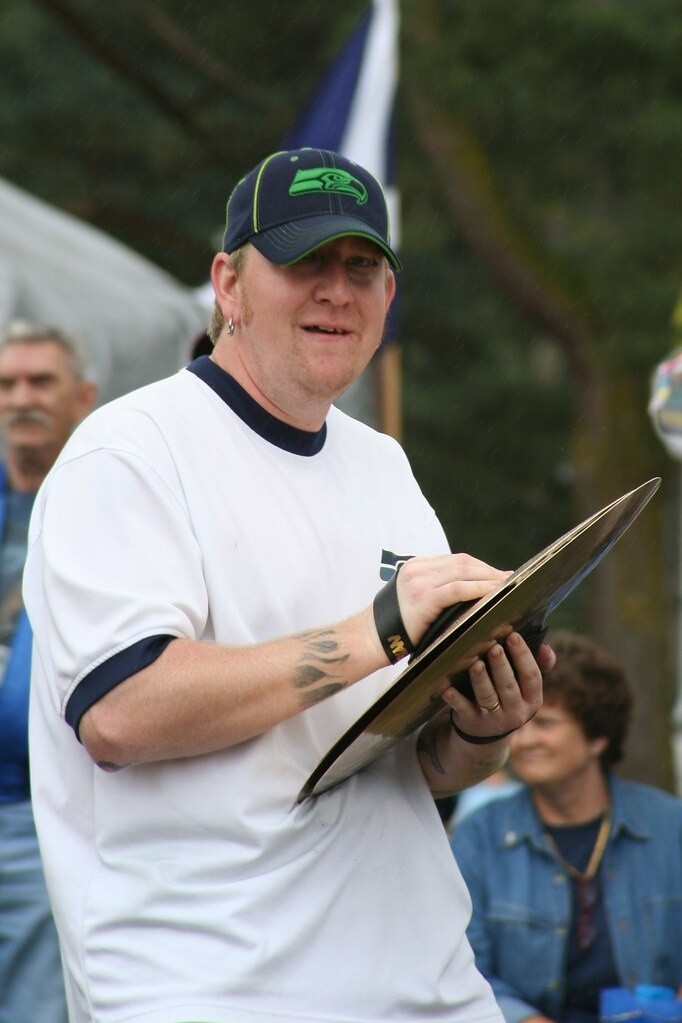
[281, 480, 667, 815]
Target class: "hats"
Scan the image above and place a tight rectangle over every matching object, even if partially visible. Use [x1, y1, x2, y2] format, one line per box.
[222, 147, 404, 275]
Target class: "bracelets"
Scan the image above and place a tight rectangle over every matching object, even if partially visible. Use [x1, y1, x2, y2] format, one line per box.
[372, 569, 416, 666]
[450, 707, 536, 744]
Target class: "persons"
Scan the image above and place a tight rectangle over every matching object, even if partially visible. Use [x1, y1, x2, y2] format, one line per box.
[0, 319, 105, 1023]
[24, 149, 506, 1023]
[448, 629, 682, 1023]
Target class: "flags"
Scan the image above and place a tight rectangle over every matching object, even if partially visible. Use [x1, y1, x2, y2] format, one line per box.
[282, 0, 402, 253]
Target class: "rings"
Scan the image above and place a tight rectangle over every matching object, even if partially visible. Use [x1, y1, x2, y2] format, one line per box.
[480, 701, 501, 713]
